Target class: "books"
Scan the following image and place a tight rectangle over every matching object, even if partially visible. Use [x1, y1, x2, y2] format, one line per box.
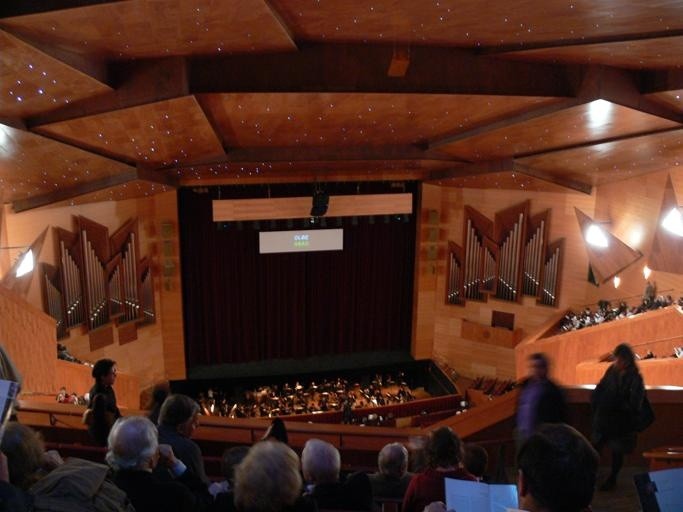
[631, 466, 683, 512]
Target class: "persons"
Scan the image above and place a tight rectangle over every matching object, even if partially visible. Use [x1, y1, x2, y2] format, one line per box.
[3, 417, 600, 512]
[588, 342, 654, 492]
[55, 385, 69, 403]
[559, 280, 683, 360]
[82, 358, 125, 446]
[186, 368, 517, 429]
[78, 391, 90, 406]
[512, 351, 570, 494]
[156, 393, 232, 504]
[0, 344, 25, 424]
[67, 392, 78, 405]
